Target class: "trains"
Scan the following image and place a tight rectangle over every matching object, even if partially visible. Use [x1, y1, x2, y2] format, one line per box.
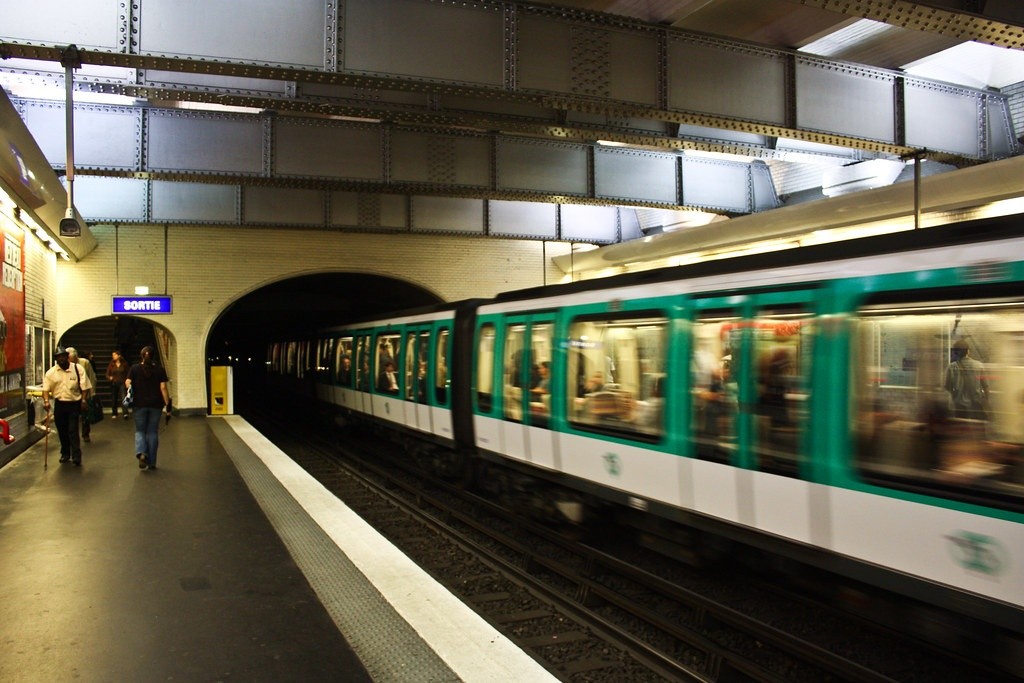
[250, 231, 1024, 637]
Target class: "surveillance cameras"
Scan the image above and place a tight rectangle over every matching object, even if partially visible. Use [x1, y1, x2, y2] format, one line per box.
[60, 218, 81, 238]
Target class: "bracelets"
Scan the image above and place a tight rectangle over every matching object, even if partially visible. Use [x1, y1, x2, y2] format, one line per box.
[81, 400, 86, 402]
[44, 400, 49, 402]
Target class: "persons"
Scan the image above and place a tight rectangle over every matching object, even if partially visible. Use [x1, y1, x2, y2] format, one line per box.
[80, 350, 97, 374]
[125, 347, 172, 471]
[65, 348, 97, 443]
[339, 340, 990, 477]
[41, 347, 93, 466]
[106, 351, 130, 418]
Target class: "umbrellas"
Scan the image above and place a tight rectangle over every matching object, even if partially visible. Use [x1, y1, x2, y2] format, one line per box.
[165, 398, 172, 425]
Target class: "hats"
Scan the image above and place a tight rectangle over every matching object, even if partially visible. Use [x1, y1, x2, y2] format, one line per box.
[53, 346, 69, 358]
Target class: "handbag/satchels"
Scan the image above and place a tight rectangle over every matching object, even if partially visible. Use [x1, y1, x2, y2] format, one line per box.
[84, 394, 104, 424]
[122, 383, 134, 413]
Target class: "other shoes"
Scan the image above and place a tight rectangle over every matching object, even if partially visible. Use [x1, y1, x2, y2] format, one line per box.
[139, 454, 147, 469]
[59, 454, 69, 462]
[84, 436, 90, 442]
[73, 456, 81, 465]
[112, 415, 116, 419]
[149, 463, 156, 469]
[124, 414, 128, 419]
